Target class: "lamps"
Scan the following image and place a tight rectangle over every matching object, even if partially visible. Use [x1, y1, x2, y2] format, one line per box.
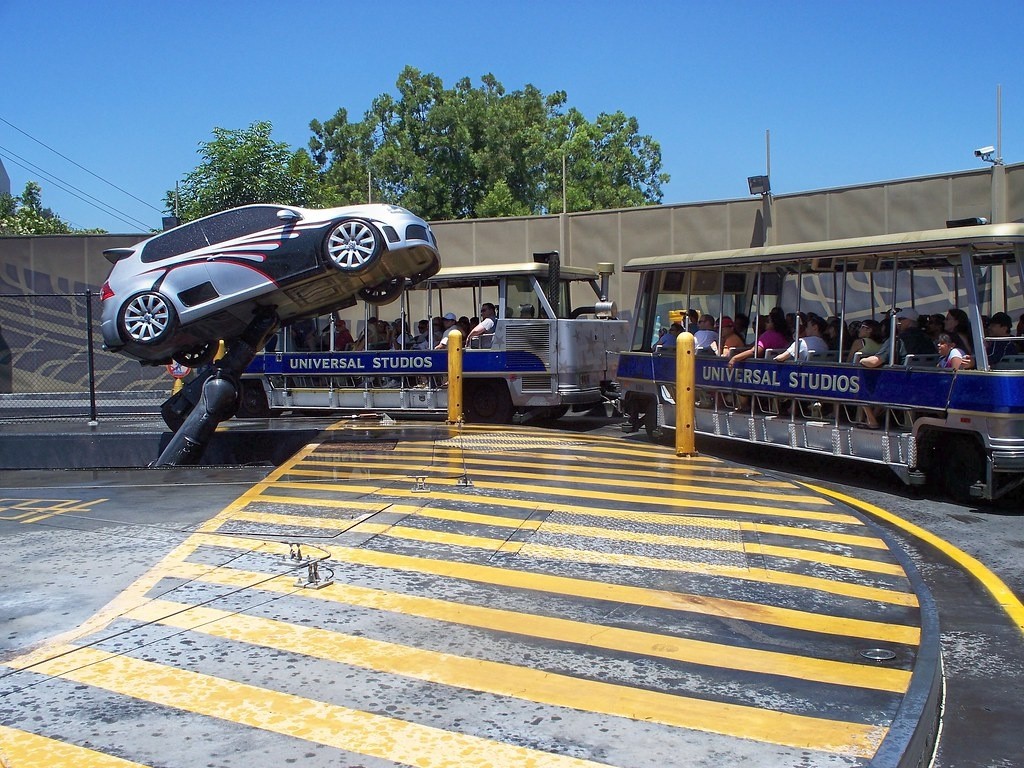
[748, 176, 770, 196]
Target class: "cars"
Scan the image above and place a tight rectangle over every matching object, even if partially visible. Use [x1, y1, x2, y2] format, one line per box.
[100, 202, 443, 368]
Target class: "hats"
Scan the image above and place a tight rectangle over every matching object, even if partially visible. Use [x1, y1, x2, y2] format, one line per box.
[881, 307, 902, 314]
[896, 307, 919, 322]
[335, 320, 346, 326]
[714, 316, 733, 329]
[680, 309, 699, 318]
[367, 317, 377, 321]
[519, 304, 535, 313]
[988, 312, 1013, 327]
[442, 313, 456, 321]
[391, 318, 402, 326]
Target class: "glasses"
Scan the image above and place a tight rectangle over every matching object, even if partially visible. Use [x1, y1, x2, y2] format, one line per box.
[699, 320, 706, 324]
[766, 319, 773, 324]
[433, 323, 440, 326]
[417, 326, 427, 328]
[481, 308, 487, 313]
[861, 324, 868, 328]
[669, 328, 674, 331]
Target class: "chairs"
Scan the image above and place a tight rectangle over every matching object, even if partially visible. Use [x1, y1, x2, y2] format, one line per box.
[295, 333, 493, 389]
[722, 344, 1024, 429]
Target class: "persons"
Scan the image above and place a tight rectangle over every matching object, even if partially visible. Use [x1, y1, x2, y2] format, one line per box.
[651, 306, 1024, 371]
[318, 302, 550, 351]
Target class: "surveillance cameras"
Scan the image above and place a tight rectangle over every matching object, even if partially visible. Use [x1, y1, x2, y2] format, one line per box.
[975, 146, 995, 157]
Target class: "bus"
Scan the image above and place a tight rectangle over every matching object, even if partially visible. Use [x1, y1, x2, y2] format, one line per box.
[169, 250, 628, 425]
[616, 223, 1024, 503]
